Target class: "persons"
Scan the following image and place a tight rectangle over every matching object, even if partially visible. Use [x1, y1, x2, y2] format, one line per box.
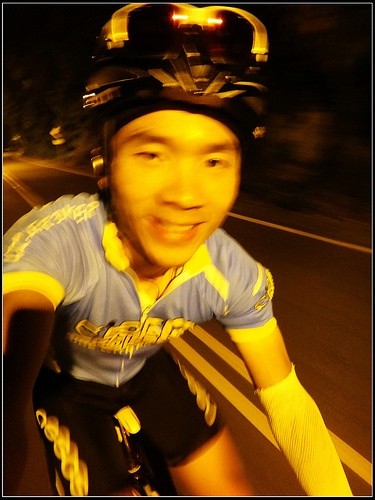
[4, 0, 356, 497]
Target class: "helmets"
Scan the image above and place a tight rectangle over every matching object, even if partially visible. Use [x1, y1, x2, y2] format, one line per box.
[81, 1, 272, 144]
